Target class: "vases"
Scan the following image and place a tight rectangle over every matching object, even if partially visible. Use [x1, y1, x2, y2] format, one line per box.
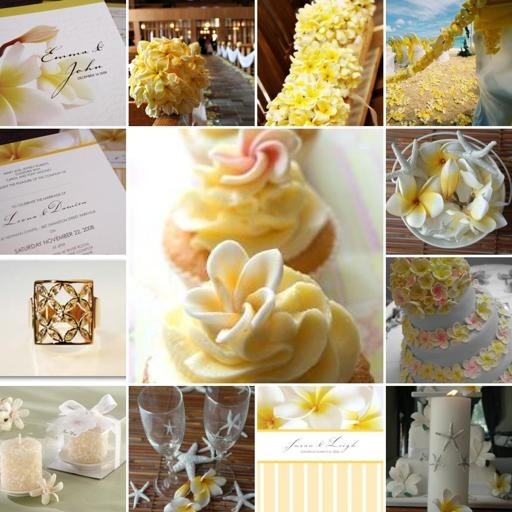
[152, 115, 185, 126]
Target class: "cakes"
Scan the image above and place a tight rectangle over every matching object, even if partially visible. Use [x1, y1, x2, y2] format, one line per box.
[388, 257, 511, 384]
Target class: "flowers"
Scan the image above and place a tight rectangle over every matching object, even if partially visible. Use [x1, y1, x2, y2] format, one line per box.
[128, 37, 212, 118]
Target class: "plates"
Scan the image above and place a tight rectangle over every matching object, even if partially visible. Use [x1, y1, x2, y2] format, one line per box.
[1, 477, 55, 499]
[57, 449, 123, 470]
[394, 144, 508, 248]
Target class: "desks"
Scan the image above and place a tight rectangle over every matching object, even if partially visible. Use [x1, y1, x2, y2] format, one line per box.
[129, 386, 254, 512]
[386, 129, 512, 255]
[0, 386, 126, 512]
[201, 54, 255, 125]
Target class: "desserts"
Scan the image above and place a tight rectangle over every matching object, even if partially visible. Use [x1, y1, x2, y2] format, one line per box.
[141, 129, 377, 384]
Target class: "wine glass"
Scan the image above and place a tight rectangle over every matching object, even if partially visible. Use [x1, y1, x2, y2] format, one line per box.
[137, 385, 251, 502]
